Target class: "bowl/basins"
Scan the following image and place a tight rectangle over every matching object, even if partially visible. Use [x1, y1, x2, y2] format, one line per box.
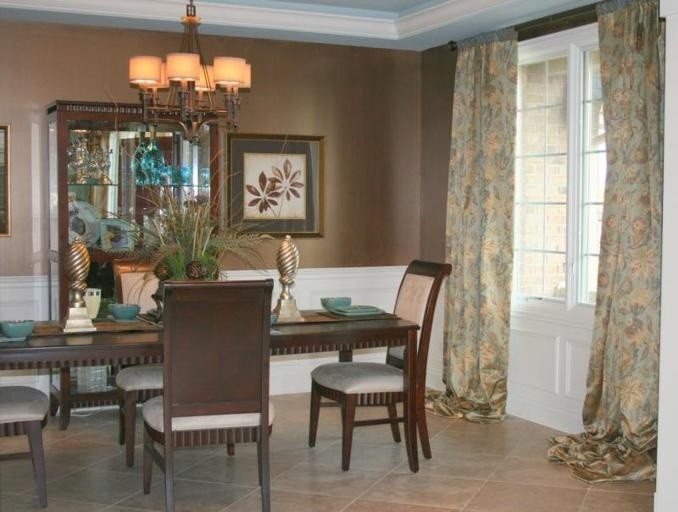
[320, 297, 352, 311]
[0, 320, 36, 339]
[108, 303, 141, 320]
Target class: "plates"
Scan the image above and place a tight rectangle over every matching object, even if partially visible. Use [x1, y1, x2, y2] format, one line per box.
[331, 305, 385, 317]
[67, 201, 102, 248]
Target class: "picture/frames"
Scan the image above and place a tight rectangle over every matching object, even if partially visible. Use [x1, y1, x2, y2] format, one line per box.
[0, 124, 11, 238]
[227, 131, 325, 238]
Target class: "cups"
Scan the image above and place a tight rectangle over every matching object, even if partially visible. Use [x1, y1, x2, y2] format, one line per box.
[85, 288, 102, 320]
[270, 314, 276, 326]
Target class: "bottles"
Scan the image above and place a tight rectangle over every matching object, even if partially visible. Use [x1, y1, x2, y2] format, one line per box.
[131, 124, 147, 185]
[142, 123, 164, 186]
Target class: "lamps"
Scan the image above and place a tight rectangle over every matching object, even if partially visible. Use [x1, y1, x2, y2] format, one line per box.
[129, 0, 252, 145]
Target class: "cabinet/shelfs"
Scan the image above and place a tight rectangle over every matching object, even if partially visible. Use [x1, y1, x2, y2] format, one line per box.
[44, 100, 230, 431]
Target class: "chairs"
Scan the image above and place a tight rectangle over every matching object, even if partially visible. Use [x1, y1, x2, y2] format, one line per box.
[0, 385, 50, 508]
[141, 280, 274, 511]
[112, 260, 163, 468]
[309, 260, 452, 470]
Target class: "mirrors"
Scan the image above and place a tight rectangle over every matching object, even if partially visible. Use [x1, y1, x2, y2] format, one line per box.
[66, 121, 211, 250]
